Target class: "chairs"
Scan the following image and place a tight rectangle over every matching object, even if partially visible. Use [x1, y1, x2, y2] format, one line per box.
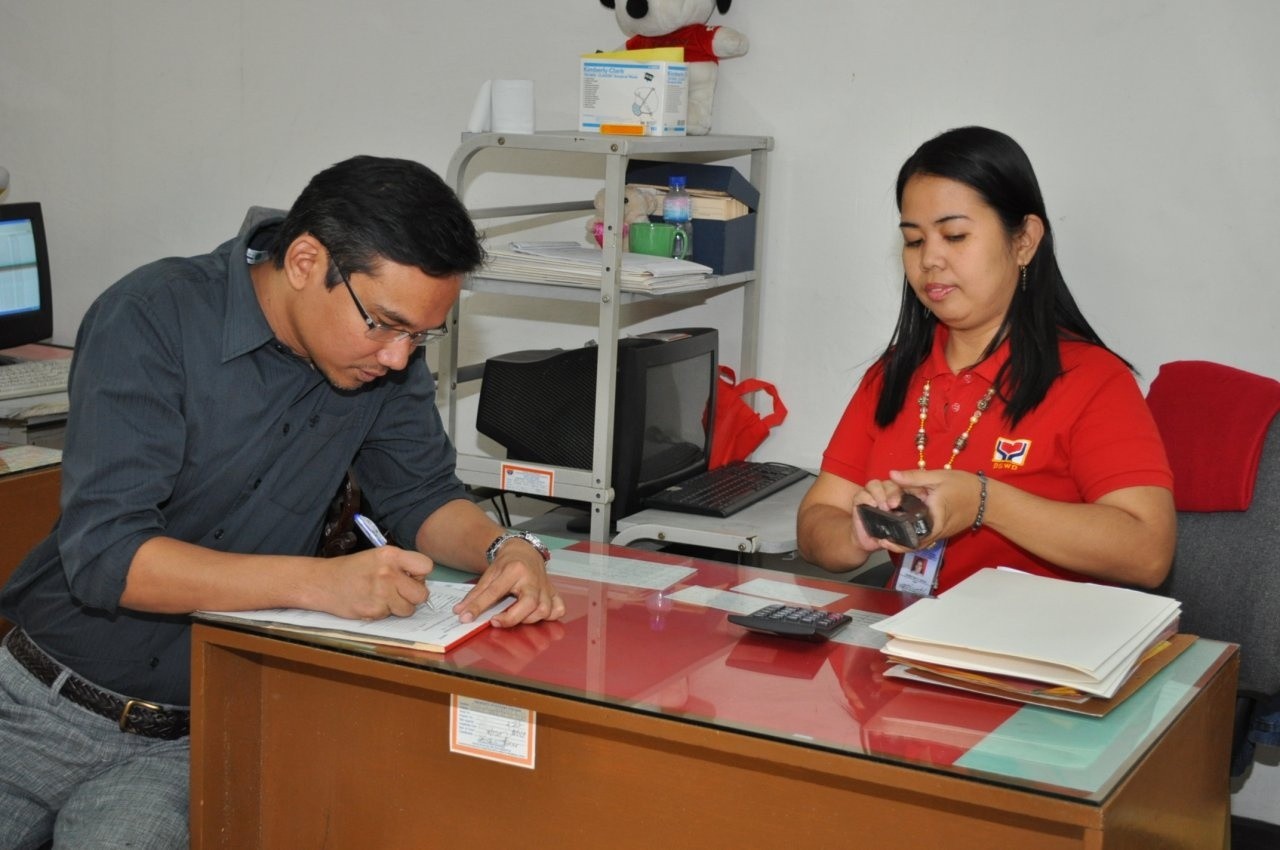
[1120, 359, 1279, 778]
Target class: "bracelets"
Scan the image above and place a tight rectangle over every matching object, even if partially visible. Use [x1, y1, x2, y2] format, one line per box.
[970, 470, 988, 531]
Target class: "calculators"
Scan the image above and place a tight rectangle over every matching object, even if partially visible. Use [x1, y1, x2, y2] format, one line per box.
[727, 603, 853, 642]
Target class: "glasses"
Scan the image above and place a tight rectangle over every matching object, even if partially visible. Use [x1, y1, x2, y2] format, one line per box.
[307, 230, 449, 347]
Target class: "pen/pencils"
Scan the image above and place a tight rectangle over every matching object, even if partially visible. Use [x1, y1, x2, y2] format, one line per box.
[353, 512, 435, 612]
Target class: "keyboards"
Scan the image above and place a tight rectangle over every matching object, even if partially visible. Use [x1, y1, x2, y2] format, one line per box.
[1, 356, 72, 401]
[643, 459, 809, 518]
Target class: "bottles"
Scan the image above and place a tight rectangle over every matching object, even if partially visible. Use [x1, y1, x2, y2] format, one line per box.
[663, 176, 694, 262]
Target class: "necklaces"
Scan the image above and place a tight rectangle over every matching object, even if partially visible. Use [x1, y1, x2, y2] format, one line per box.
[915, 377, 998, 472]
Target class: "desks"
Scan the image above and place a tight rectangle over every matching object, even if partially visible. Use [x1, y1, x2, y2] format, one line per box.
[0, 343, 79, 639]
[190, 532, 1244, 849]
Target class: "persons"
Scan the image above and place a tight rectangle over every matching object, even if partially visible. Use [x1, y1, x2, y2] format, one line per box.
[793, 124, 1178, 597]
[1, 151, 569, 850]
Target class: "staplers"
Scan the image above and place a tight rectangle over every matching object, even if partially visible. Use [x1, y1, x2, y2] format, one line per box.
[857, 491, 935, 550]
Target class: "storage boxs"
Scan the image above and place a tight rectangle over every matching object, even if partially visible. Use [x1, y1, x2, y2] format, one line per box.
[582, 59, 687, 138]
[625, 157, 760, 279]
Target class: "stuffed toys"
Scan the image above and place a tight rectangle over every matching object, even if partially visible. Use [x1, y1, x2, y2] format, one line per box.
[585, 185, 659, 254]
[596, 0, 751, 136]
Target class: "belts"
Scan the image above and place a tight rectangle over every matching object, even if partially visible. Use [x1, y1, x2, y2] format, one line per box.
[7, 628, 190, 740]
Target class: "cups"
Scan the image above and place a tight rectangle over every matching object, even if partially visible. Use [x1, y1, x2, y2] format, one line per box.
[628, 222, 688, 259]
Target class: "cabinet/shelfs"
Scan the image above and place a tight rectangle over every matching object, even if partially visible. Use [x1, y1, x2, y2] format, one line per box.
[436, 126, 781, 547]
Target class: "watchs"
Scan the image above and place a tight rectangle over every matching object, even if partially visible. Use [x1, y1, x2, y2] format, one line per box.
[485, 527, 552, 575]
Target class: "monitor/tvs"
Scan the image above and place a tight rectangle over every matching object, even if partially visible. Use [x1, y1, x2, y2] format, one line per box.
[0, 202, 54, 365]
[475, 327, 719, 522]
